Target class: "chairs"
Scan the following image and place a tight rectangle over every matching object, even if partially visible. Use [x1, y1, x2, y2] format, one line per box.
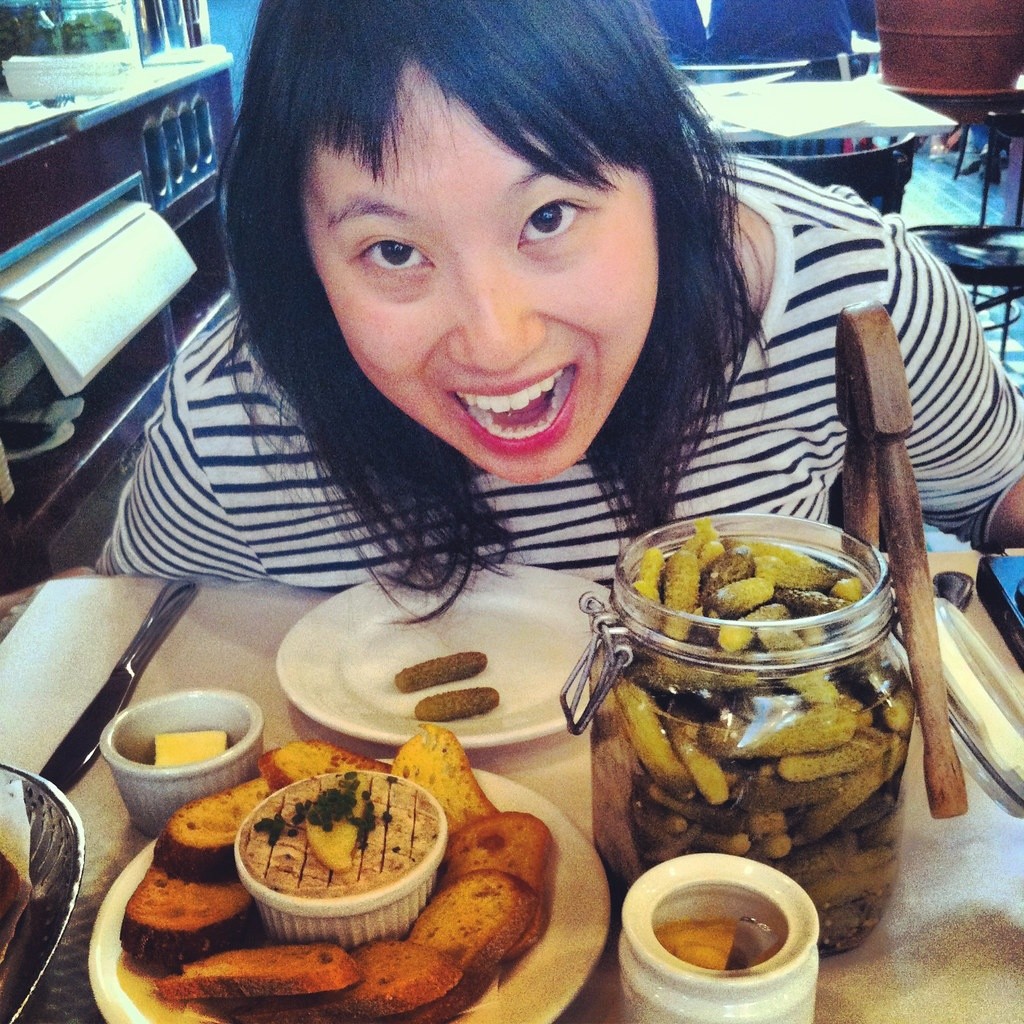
[904, 111, 1024, 366]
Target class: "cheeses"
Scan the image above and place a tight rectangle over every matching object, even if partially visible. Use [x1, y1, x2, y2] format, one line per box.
[153, 730, 231, 766]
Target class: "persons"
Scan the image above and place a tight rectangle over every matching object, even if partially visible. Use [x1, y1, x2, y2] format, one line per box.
[979, 127, 1010, 190]
[706, 0, 853, 65]
[0, 0, 1024, 621]
[647, 0, 707, 65]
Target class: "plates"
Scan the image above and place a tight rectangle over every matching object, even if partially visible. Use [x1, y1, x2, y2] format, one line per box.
[276, 564, 639, 749]
[86, 755, 611, 1024]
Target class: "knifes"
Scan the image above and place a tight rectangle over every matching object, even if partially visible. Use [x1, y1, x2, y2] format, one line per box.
[38, 579, 200, 792]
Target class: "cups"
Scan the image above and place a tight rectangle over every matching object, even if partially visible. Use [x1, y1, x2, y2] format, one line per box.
[99, 689, 265, 839]
[232, 769, 447, 947]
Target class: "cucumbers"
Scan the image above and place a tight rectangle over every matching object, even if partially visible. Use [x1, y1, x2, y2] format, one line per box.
[588, 517, 916, 958]
[395, 651, 499, 723]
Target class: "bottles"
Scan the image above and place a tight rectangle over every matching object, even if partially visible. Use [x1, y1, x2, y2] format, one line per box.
[618, 851, 818, 1023]
[562, 513, 917, 958]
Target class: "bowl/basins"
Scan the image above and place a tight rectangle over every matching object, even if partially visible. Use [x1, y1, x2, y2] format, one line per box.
[0, 761, 86, 1024]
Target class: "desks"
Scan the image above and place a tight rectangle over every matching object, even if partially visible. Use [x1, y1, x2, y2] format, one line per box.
[1, 560, 1024, 1024]
[750, 129, 926, 219]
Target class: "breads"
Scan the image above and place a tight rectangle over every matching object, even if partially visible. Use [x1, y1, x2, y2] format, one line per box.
[121, 723, 553, 1024]
[0, 850, 19, 923]
